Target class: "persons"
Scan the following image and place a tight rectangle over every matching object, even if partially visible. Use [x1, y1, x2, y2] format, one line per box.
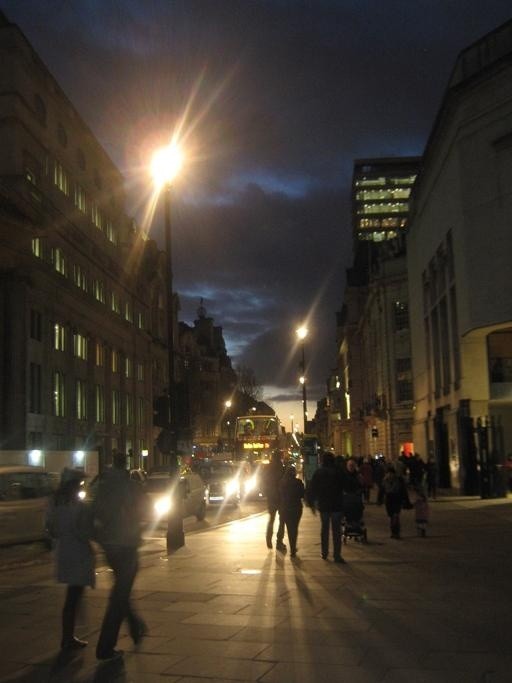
[238, 422, 255, 438]
[46, 467, 98, 650]
[256, 446, 441, 566]
[91, 452, 150, 659]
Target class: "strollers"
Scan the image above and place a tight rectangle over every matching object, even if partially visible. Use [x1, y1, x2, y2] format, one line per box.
[342, 493, 367, 543]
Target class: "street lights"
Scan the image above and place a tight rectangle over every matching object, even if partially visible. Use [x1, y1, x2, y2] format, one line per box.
[297, 323, 308, 433]
[147, 140, 185, 547]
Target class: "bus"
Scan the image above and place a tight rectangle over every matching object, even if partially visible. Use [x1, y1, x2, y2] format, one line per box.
[233, 415, 288, 461]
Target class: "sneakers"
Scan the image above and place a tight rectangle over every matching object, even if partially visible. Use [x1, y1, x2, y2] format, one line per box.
[265, 539, 297, 558]
[97, 648, 123, 662]
[321, 552, 347, 565]
[62, 636, 88, 649]
[132, 621, 144, 642]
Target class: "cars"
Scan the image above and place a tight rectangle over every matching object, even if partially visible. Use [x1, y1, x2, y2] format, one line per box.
[1, 465, 205, 545]
[199, 455, 304, 507]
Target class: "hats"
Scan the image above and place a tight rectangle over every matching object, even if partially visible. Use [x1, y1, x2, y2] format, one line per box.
[60, 468, 86, 482]
